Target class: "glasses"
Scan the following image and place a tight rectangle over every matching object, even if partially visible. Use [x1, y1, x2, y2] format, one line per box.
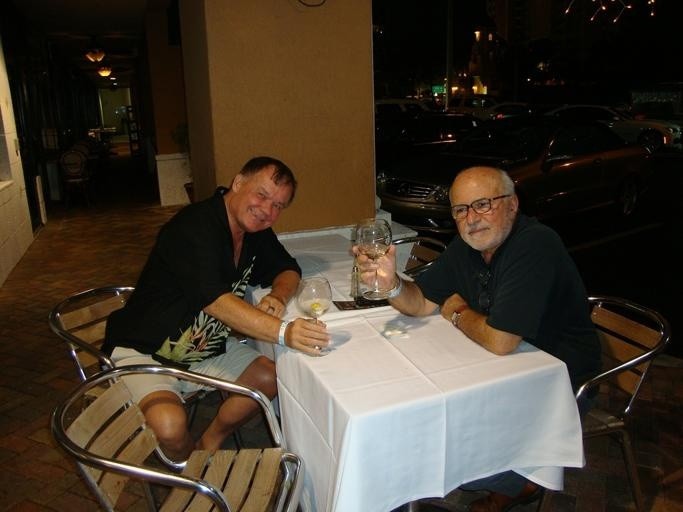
[450, 194, 513, 219]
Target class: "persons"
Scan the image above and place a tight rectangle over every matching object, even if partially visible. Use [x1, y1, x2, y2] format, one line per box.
[101, 156, 331, 508]
[352, 166, 601, 512]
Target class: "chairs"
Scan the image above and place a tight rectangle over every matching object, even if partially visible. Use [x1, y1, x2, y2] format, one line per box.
[48, 284, 246, 450]
[519, 129, 571, 150]
[56, 130, 116, 206]
[538, 294, 670, 511]
[48, 362, 305, 512]
[391, 237, 446, 275]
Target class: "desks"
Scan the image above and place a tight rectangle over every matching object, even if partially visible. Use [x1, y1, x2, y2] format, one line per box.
[243, 235, 565, 511]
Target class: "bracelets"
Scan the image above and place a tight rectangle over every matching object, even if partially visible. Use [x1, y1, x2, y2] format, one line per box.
[278, 320, 292, 347]
[385, 272, 404, 297]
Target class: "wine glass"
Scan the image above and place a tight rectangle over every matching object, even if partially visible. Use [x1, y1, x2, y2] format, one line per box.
[356, 218, 392, 301]
[297, 276, 332, 356]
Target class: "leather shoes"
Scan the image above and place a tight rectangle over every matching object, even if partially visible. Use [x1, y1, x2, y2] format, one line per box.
[469, 482, 543, 512]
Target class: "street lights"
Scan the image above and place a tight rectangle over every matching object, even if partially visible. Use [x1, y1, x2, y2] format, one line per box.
[473, 29, 493, 87]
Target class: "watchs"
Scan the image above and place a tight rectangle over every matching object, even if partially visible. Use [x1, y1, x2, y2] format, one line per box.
[451, 303, 472, 328]
